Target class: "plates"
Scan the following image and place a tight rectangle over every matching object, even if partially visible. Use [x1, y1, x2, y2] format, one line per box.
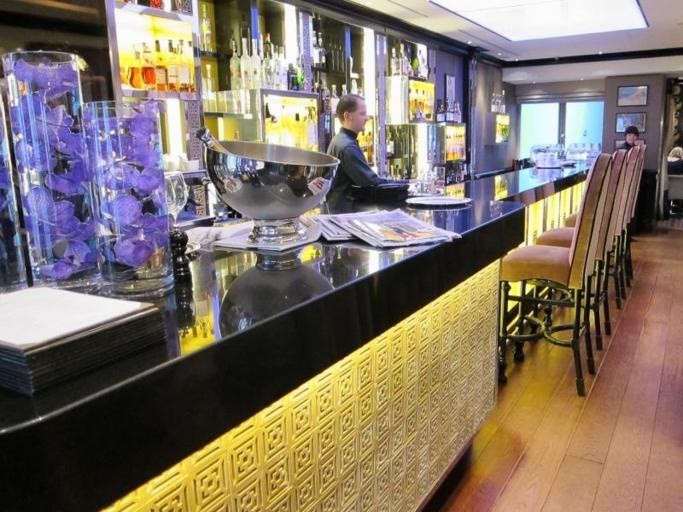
[407, 198, 471, 209]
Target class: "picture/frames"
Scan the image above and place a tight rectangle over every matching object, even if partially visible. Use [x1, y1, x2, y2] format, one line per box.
[616, 85, 648, 107]
[614, 139, 645, 152]
[615, 113, 646, 133]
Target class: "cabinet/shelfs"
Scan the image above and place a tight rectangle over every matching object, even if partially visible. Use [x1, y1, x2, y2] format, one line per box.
[100, 0, 468, 229]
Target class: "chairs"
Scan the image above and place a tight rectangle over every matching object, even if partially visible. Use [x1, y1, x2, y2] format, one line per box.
[499, 144, 646, 397]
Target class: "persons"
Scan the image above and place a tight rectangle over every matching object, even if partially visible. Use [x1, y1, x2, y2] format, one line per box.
[666, 145, 682, 162]
[324, 94, 417, 200]
[613, 126, 638, 152]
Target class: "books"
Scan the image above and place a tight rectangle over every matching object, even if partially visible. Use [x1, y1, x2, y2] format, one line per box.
[296, 205, 465, 250]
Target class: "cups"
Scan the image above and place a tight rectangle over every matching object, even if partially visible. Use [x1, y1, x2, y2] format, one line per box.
[0, 49, 100, 295]
[80, 97, 174, 300]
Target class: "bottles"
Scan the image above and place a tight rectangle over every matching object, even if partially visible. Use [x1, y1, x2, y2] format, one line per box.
[491, 93, 506, 113]
[385, 43, 469, 185]
[118, 32, 359, 156]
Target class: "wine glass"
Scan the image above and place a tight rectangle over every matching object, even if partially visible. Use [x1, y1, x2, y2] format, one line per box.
[165, 171, 189, 233]
